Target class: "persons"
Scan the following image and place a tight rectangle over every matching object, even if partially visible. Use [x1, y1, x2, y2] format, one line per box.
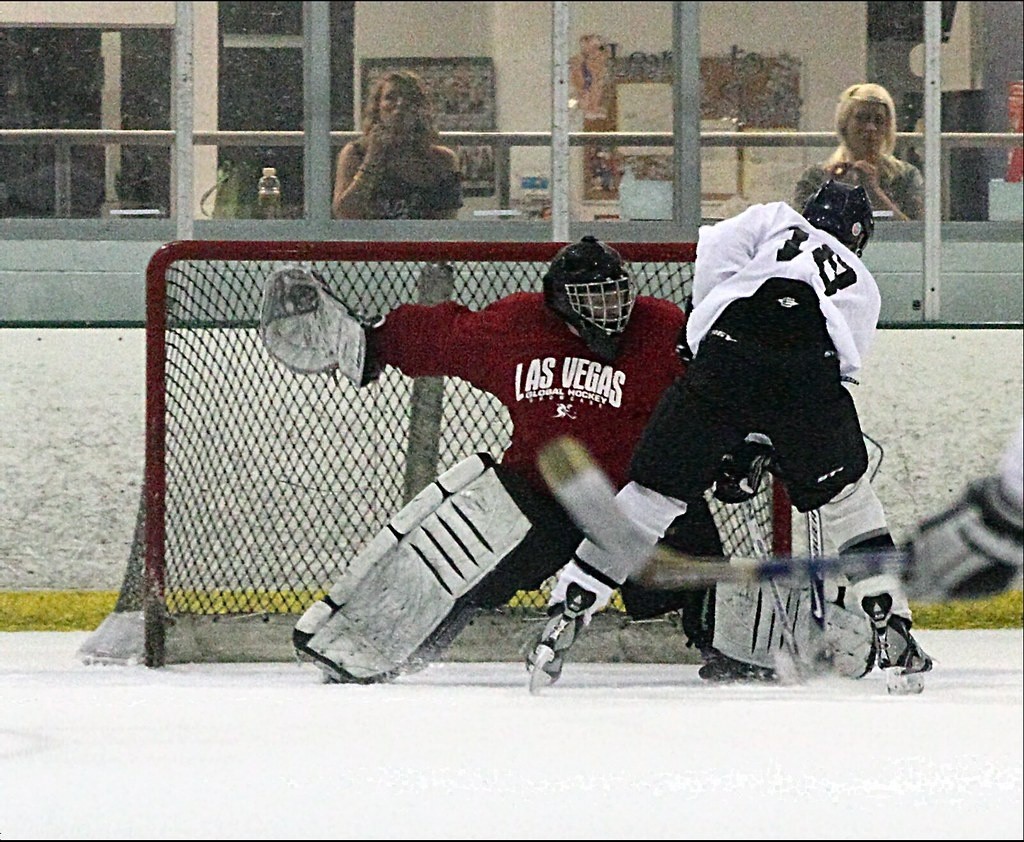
[259, 230, 783, 680]
[521, 177, 934, 680]
[788, 79, 992, 223]
[328, 68, 464, 221]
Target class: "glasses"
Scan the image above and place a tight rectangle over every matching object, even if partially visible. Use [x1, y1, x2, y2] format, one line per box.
[848, 112, 890, 125]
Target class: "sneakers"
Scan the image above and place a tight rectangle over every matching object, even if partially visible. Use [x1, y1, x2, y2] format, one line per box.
[525, 583, 596, 693]
[854, 593, 932, 696]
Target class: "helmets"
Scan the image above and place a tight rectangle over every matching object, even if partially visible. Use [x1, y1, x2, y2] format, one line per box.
[543, 235, 629, 361]
[799, 179, 874, 258]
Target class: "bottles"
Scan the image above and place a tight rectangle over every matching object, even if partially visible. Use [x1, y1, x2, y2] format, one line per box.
[257, 167, 281, 219]
[216, 155, 238, 219]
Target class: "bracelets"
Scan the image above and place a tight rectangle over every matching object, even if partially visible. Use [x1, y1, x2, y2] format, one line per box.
[351, 158, 376, 184]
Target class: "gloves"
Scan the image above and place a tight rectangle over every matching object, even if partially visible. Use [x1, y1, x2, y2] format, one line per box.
[714, 433, 774, 504]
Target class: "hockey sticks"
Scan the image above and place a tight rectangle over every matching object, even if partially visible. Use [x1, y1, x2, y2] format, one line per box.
[736, 488, 819, 693]
[531, 426, 926, 602]
